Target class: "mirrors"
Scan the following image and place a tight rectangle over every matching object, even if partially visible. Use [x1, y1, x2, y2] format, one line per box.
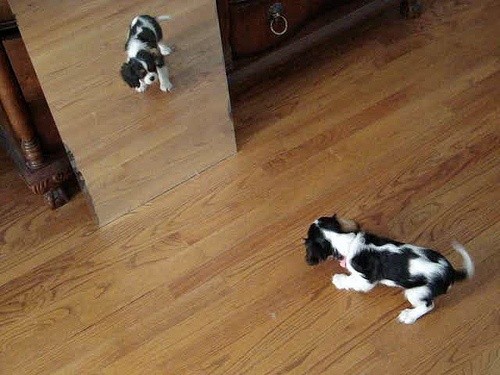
[7, 1, 238, 230]
